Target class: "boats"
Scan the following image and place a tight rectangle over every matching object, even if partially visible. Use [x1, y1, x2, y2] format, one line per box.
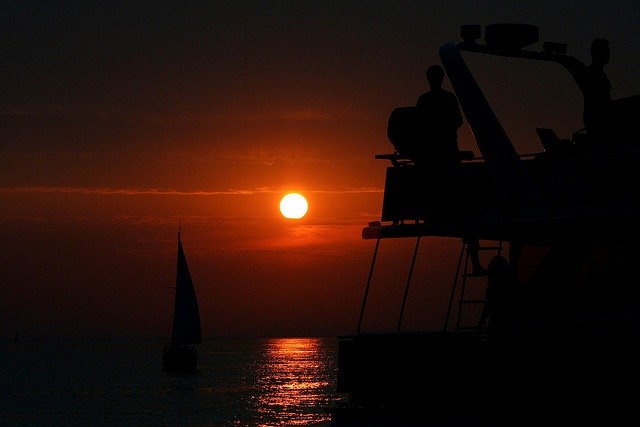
[332, 18, 635, 405]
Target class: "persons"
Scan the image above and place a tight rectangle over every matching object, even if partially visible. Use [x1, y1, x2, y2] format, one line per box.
[475, 256, 510, 332]
[468, 240, 487, 274]
[416, 65, 463, 162]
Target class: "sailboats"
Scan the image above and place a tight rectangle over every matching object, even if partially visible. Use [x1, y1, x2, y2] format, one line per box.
[161, 215, 204, 375]
